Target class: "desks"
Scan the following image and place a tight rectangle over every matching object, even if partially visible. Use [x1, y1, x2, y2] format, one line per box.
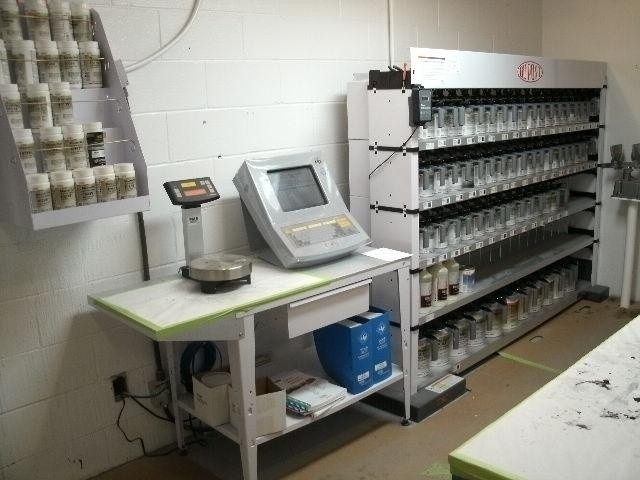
[87, 244, 412, 479]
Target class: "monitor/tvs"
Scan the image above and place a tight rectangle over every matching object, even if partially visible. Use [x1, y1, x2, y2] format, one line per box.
[263, 166, 331, 215]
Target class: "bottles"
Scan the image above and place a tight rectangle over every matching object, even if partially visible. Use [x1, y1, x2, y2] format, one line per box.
[1, 0, 138, 213]
[446, 258, 460, 295]
[419, 268, 433, 307]
[430, 262, 448, 307]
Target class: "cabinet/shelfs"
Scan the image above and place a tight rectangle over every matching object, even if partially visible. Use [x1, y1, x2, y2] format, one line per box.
[346, 47, 609, 419]
[0, 7, 150, 229]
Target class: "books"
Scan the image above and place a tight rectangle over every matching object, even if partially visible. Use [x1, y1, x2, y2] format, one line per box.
[271, 364, 348, 419]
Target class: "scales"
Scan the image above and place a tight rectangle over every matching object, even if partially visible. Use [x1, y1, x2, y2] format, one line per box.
[163, 177, 252, 294]
